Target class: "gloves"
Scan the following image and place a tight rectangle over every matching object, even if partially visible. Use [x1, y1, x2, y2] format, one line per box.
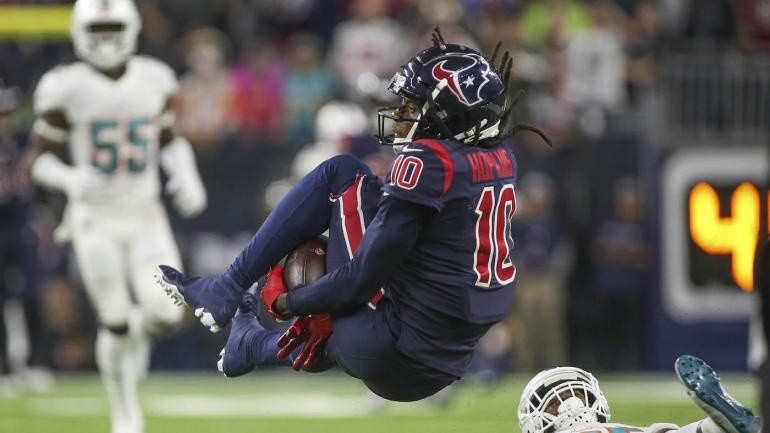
[159, 136, 207, 217]
[275, 313, 333, 371]
[30, 149, 103, 205]
[259, 262, 290, 323]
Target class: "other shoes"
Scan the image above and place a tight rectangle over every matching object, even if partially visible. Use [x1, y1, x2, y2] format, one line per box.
[103, 384, 146, 433]
[675, 354, 763, 433]
[217, 291, 265, 379]
[153, 265, 238, 333]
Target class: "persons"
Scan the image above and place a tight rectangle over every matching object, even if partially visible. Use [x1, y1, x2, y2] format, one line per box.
[517, 354, 770, 433]
[0, 0, 770, 433]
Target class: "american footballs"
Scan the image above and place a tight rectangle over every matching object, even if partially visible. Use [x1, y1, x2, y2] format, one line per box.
[284, 233, 326, 292]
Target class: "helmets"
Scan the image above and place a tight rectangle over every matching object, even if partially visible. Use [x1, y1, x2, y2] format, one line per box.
[70, 0, 142, 70]
[313, 101, 370, 144]
[516, 364, 611, 433]
[384, 44, 505, 142]
